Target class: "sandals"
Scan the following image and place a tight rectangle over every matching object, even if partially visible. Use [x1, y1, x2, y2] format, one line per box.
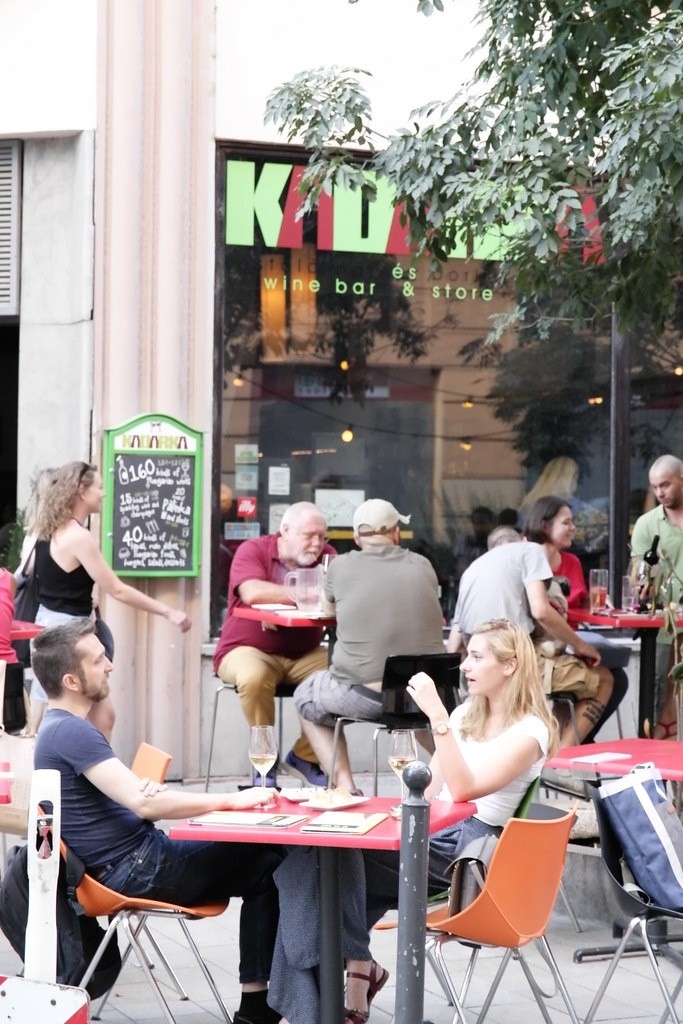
[344, 960, 389, 1024]
[654, 721, 677, 741]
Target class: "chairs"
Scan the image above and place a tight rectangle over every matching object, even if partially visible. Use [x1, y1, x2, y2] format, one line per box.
[325, 653, 683, 1024]
[37, 804, 231, 1024]
[206, 608, 293, 793]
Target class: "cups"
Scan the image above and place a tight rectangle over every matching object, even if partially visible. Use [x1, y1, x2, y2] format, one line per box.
[588, 565, 610, 611]
[618, 569, 642, 617]
[285, 565, 327, 613]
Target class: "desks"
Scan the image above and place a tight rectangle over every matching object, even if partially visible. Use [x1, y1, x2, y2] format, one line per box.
[170, 796, 479, 1024]
[234, 607, 447, 668]
[544, 738, 683, 964]
[566, 608, 683, 739]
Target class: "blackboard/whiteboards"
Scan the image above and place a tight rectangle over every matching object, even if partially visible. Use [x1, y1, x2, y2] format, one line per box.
[103, 449, 202, 578]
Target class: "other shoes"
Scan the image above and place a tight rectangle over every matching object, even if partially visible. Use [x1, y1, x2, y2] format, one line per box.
[234, 1012, 290, 1024]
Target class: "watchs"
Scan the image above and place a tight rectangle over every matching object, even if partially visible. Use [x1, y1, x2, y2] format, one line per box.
[431, 722, 452, 736]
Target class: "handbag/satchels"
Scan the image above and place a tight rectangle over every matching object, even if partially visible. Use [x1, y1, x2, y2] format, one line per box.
[10, 547, 38, 667]
[443, 833, 498, 918]
[598, 761, 683, 910]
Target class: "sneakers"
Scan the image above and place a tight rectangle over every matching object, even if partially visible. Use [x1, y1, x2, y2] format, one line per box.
[254, 753, 280, 787]
[540, 766, 595, 797]
[282, 751, 336, 790]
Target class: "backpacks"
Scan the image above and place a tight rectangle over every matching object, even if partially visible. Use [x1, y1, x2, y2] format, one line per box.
[1, 845, 121, 1001]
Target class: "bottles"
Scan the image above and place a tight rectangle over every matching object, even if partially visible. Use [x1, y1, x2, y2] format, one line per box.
[637, 534, 661, 592]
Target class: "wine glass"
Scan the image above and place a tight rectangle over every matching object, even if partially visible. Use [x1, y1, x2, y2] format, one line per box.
[387, 725, 419, 817]
[245, 720, 279, 806]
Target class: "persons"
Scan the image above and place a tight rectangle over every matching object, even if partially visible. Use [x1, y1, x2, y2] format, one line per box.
[523, 496, 629, 743]
[517, 456, 610, 553]
[630, 454, 683, 739]
[446, 526, 613, 751]
[267, 618, 561, 1024]
[293, 498, 445, 796]
[0, 566, 19, 664]
[31, 619, 290, 1024]
[451, 507, 517, 580]
[213, 501, 338, 788]
[12, 460, 192, 741]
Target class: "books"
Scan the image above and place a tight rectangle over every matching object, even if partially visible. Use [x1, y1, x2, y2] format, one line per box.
[300, 810, 388, 835]
[189, 810, 309, 829]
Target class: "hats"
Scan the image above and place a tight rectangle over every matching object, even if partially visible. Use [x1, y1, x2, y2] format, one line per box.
[354, 498, 412, 537]
